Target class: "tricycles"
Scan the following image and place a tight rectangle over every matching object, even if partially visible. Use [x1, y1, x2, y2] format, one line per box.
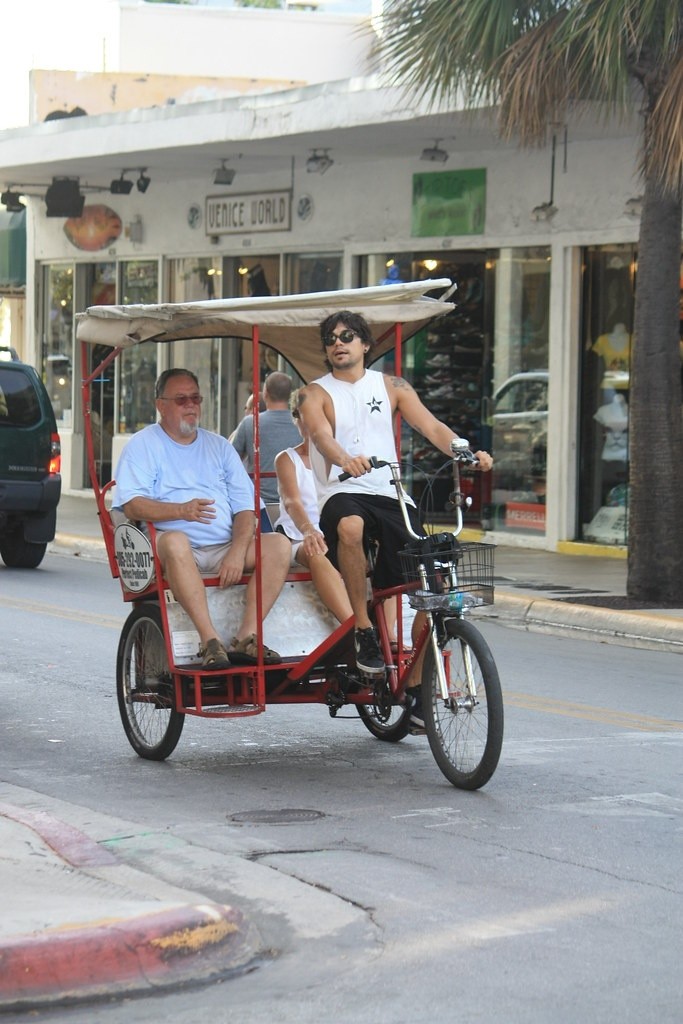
[73, 274, 505, 792]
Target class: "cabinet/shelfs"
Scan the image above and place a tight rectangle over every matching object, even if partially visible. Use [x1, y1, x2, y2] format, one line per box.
[412, 340, 484, 481]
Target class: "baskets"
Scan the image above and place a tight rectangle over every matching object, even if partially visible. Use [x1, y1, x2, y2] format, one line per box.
[394, 541, 498, 613]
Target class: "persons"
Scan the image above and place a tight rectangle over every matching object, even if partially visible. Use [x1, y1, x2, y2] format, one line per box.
[229, 392, 267, 470]
[593, 323, 630, 389]
[295, 310, 494, 729]
[274, 385, 413, 654]
[593, 394, 629, 488]
[228, 371, 303, 503]
[110, 368, 292, 671]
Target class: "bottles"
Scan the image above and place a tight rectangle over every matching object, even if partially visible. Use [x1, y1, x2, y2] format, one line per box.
[414, 589, 483, 611]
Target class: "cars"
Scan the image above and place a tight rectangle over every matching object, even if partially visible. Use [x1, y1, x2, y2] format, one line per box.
[486, 368, 630, 499]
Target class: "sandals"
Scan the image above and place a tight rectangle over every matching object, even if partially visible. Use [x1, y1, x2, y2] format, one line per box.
[225, 632, 283, 666]
[197, 637, 233, 669]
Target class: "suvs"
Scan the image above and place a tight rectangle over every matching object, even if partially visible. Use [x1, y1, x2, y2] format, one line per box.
[0, 345, 62, 568]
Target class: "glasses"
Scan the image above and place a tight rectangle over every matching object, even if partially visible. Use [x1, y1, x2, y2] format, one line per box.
[291, 409, 301, 418]
[158, 393, 205, 407]
[323, 329, 358, 347]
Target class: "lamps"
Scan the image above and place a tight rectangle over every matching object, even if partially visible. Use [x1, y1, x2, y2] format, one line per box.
[214, 158, 235, 185]
[1, 165, 150, 218]
[529, 123, 564, 222]
[621, 110, 651, 225]
[305, 148, 333, 175]
[420, 138, 448, 162]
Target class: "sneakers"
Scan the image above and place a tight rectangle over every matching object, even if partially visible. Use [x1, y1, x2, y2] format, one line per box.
[354, 623, 387, 674]
[405, 683, 427, 729]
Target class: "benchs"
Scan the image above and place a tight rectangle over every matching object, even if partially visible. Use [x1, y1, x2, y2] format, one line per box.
[97, 471, 375, 603]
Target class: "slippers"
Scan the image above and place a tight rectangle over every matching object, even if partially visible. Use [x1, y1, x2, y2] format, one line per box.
[388, 641, 413, 654]
